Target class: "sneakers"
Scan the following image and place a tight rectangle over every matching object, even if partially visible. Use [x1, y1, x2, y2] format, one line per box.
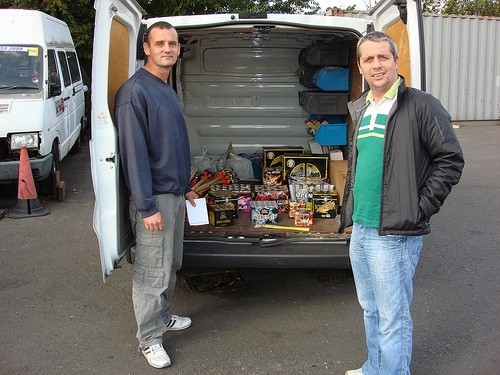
[141, 344, 171, 368]
[166, 315, 192, 330]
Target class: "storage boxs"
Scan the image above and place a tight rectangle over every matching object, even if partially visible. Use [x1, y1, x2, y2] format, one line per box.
[207, 38, 354, 228]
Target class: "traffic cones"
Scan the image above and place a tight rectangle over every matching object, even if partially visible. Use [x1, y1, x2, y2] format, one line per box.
[5, 146, 51, 218]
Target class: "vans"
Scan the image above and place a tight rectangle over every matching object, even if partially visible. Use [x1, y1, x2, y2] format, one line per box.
[86, 0, 429, 285]
[0, 9, 89, 194]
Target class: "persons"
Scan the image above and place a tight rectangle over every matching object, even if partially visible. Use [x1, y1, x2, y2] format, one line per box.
[112, 22, 201, 368]
[337, 32, 463, 375]
